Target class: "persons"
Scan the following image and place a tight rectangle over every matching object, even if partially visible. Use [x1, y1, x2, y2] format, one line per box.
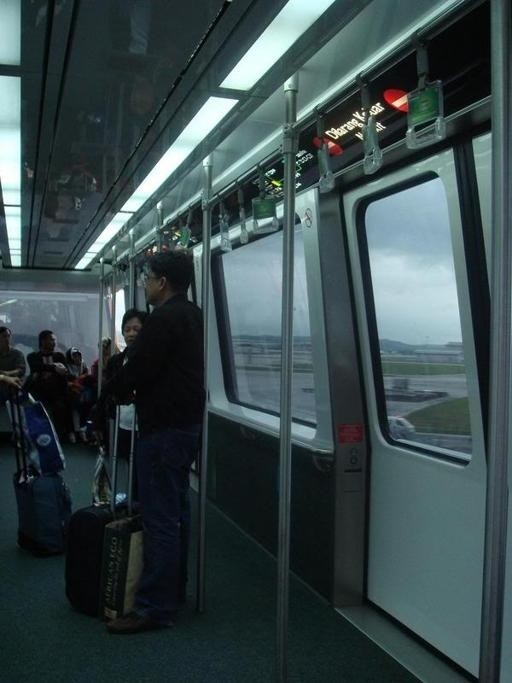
[106, 249, 207, 633]
[94, 308, 149, 500]
[22, 325, 122, 454]
[0, 326, 26, 409]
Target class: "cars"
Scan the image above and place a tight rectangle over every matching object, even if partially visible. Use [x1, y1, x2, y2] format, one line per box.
[385, 414, 417, 435]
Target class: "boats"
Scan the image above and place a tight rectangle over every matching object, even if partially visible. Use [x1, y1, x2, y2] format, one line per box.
[300, 376, 450, 402]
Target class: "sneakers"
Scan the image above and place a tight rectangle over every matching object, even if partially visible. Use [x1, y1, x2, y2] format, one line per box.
[106, 609, 174, 633]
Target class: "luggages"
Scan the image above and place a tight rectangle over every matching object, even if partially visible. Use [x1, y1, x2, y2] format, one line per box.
[66, 390, 137, 616]
[9, 387, 72, 556]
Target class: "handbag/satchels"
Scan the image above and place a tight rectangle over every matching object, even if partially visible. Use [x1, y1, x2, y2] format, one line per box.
[6, 391, 66, 477]
[92, 454, 128, 508]
[99, 515, 144, 621]
